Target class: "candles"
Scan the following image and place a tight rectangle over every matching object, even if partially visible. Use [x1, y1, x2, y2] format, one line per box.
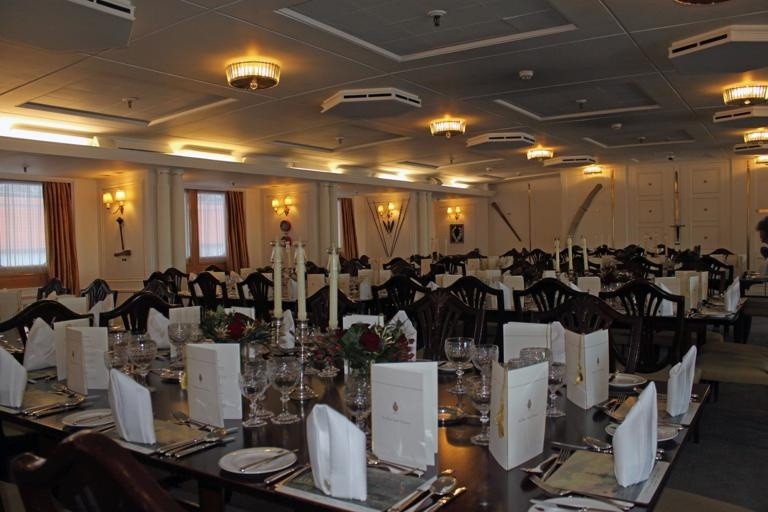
[581, 237, 589, 271]
[554, 239, 560, 273]
[567, 237, 574, 270]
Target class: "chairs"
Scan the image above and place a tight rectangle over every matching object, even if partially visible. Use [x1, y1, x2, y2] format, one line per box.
[1, 423, 58, 510]
[0, 244, 751, 374]
[51, 431, 185, 512]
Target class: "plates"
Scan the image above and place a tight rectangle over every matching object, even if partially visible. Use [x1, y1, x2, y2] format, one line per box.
[436, 405, 464, 424]
[219, 446, 297, 475]
[603, 422, 680, 444]
[64, 408, 112, 428]
[605, 372, 647, 388]
[527, 498, 621, 512]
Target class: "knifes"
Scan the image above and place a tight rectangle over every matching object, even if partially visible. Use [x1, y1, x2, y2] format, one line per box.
[263, 463, 307, 486]
[157, 426, 238, 457]
[530, 499, 594, 512]
[73, 411, 113, 425]
[386, 468, 454, 511]
[422, 486, 465, 512]
[608, 369, 624, 381]
[240, 447, 301, 472]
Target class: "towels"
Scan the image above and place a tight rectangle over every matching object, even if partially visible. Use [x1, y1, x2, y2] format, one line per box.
[305, 401, 370, 504]
[0, 347, 30, 409]
[666, 346, 699, 416]
[105, 367, 158, 448]
[611, 382, 657, 489]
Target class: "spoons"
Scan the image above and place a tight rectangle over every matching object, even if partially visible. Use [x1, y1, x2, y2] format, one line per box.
[631, 387, 699, 402]
[405, 476, 457, 512]
[582, 435, 664, 459]
[171, 410, 218, 432]
[365, 451, 425, 476]
[21, 396, 85, 417]
[50, 381, 78, 398]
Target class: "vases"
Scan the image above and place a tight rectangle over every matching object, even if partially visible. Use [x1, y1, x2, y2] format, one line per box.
[348, 377, 371, 434]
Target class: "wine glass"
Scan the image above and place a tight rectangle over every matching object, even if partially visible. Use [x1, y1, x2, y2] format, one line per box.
[110, 323, 190, 390]
[447, 339, 565, 447]
[600, 280, 624, 310]
[344, 370, 372, 431]
[238, 357, 301, 425]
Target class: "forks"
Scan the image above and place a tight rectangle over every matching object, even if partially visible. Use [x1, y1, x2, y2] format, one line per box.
[528, 476, 635, 512]
[543, 445, 571, 481]
[591, 391, 627, 416]
[518, 451, 558, 475]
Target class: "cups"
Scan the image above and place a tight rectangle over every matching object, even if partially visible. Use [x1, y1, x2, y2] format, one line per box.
[216, 273, 359, 302]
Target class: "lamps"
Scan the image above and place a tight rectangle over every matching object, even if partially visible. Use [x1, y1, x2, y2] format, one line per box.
[742, 130, 768, 143]
[722, 85, 768, 107]
[755, 155, 768, 164]
[446, 205, 462, 219]
[583, 165, 603, 175]
[225, 59, 280, 93]
[376, 202, 394, 216]
[527, 144, 554, 163]
[99, 189, 131, 217]
[429, 114, 466, 141]
[271, 195, 293, 216]
